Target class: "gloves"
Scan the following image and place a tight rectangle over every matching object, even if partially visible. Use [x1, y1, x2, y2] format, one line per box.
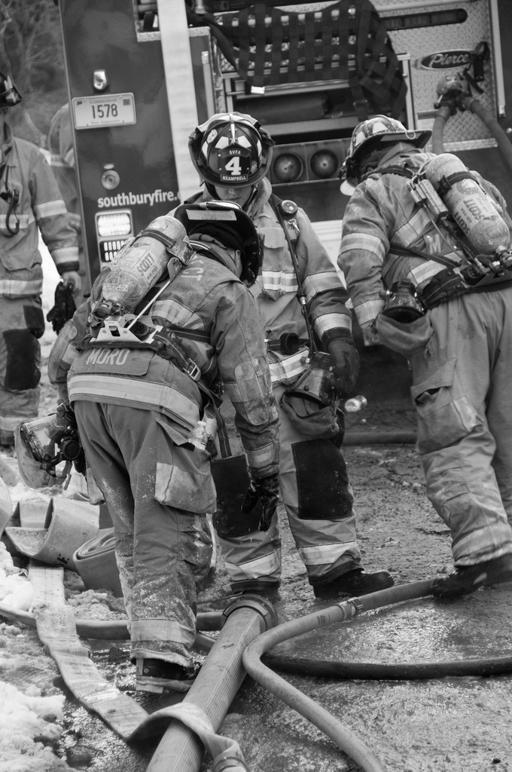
[326, 337, 362, 394]
[253, 474, 279, 530]
[47, 281, 77, 335]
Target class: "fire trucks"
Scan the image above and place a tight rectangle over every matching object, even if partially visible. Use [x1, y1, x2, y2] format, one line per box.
[59, 0, 511, 382]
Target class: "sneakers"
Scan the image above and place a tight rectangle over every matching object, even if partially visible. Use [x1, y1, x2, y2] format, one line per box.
[133, 655, 202, 694]
[313, 568, 393, 598]
[241, 590, 282, 605]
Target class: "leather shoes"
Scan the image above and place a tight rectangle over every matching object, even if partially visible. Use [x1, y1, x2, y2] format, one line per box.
[431, 550, 511, 599]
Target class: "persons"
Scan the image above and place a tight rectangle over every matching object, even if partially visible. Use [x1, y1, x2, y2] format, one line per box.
[167, 112, 393, 616]
[334, 112, 512, 602]
[0, 69, 86, 449]
[48, 101, 94, 310]
[50, 198, 286, 698]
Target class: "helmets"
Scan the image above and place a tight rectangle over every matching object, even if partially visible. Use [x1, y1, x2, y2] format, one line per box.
[336, 115, 431, 182]
[186, 110, 276, 188]
[173, 198, 261, 287]
[0, 73, 21, 107]
